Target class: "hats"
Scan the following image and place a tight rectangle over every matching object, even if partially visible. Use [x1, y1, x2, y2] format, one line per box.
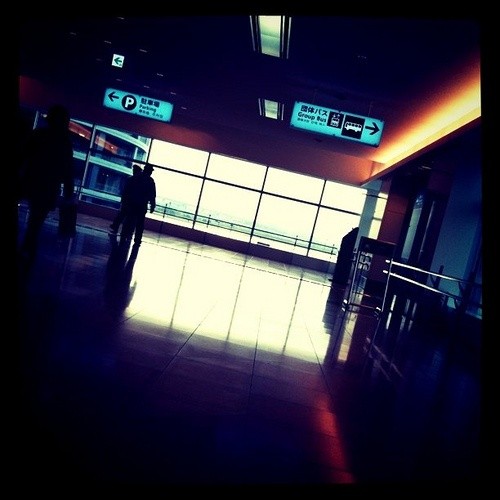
[144, 162, 154, 171]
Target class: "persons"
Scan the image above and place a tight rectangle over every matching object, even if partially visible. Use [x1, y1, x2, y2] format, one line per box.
[110, 165, 147, 243]
[22, 103, 74, 266]
[121, 163, 155, 236]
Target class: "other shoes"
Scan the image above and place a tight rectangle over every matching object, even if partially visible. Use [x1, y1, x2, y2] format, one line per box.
[109, 224, 115, 230]
[134, 238, 142, 242]
[128, 235, 131, 239]
[120, 233, 124, 236]
